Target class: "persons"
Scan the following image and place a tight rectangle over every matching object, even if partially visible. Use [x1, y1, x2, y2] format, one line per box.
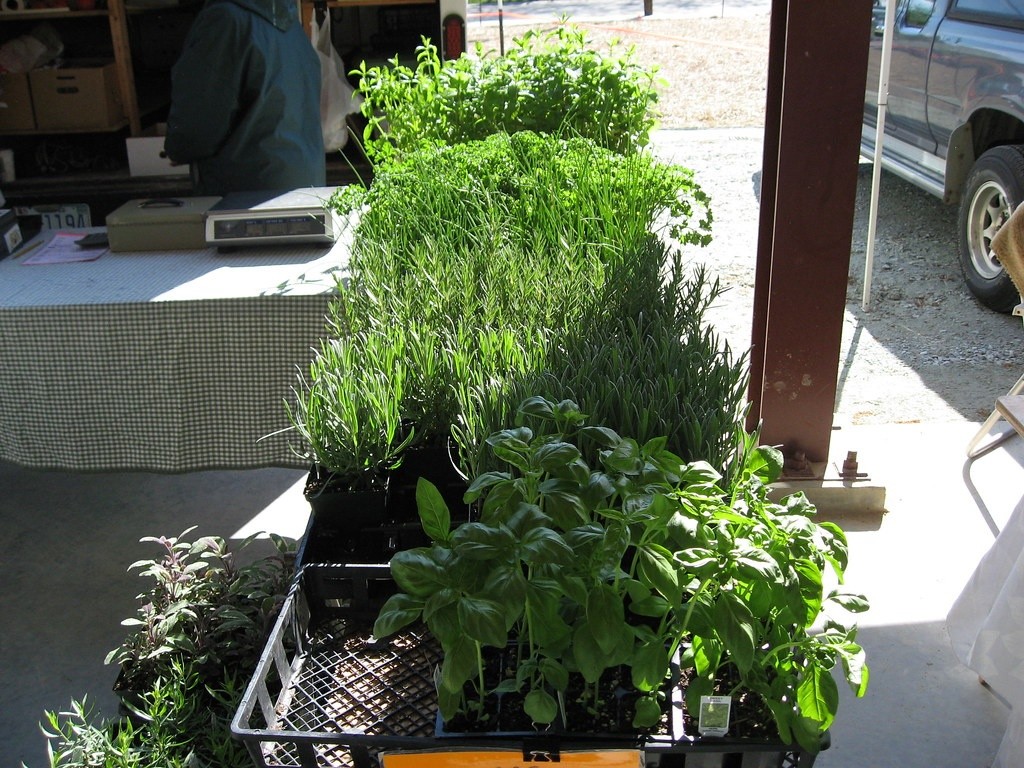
[160, 1, 327, 197]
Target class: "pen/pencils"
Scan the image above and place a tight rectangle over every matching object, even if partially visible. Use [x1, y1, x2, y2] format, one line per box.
[11, 240, 45, 259]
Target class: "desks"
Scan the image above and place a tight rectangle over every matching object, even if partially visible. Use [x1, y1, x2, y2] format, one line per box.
[0, 225, 358, 475]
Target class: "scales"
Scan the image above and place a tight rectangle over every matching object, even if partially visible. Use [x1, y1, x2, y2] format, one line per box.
[203, 184, 353, 252]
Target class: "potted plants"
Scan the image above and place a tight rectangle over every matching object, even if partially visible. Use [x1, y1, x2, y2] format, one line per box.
[19, 18, 874, 768]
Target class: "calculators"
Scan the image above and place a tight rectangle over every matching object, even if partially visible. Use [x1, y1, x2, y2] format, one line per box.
[73, 232, 109, 250]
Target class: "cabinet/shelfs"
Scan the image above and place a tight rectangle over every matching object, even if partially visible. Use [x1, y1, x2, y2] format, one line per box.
[0, 0, 467, 203]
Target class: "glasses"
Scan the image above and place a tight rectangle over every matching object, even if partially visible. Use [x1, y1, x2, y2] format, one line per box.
[138, 198, 184, 209]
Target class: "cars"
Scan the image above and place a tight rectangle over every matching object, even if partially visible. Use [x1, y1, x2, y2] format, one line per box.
[861, 0, 1024, 315]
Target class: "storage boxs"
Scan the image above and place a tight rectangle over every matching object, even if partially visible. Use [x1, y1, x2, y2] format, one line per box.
[0, 72, 36, 131]
[123, 122, 190, 178]
[0, 207, 24, 262]
[29, 56, 123, 129]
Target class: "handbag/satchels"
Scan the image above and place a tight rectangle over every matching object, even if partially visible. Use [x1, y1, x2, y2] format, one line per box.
[306, 5, 365, 153]
